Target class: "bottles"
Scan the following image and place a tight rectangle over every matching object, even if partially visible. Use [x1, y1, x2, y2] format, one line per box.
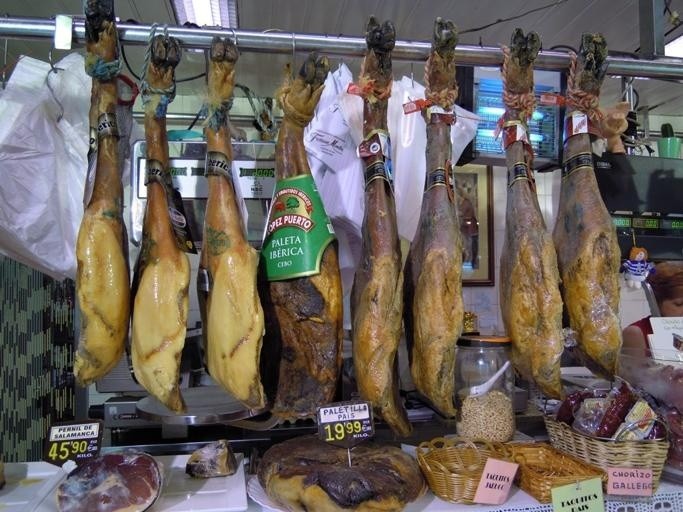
[453, 333, 517, 445]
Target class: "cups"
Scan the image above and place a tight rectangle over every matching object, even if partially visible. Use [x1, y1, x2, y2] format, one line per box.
[658, 137, 682, 158]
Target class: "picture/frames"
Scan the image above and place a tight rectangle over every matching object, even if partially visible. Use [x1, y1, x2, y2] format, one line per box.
[454, 161, 494, 287]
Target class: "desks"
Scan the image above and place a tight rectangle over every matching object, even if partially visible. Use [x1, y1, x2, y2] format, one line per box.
[23, 415, 683, 512]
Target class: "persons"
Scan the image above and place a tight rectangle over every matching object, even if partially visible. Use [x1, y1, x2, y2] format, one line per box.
[620, 261, 683, 369]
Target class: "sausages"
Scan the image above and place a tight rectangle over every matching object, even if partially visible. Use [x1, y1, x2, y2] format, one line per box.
[554, 382, 683, 461]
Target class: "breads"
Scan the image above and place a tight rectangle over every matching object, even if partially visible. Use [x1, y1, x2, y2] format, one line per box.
[56, 453, 158, 512]
[258, 432, 423, 512]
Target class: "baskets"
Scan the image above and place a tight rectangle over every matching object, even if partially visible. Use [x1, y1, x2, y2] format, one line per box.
[493, 441, 608, 503]
[415, 435, 515, 506]
[541, 414, 669, 494]
